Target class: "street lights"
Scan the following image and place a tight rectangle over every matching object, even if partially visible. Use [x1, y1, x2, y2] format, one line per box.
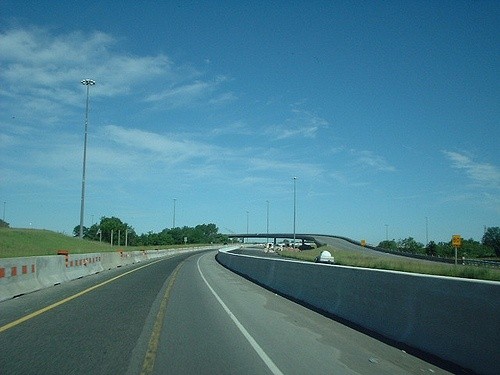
[293, 176, 297, 249]
[173, 198, 176, 228]
[246, 210, 248, 243]
[79, 78, 96, 240]
[266, 200, 269, 243]
